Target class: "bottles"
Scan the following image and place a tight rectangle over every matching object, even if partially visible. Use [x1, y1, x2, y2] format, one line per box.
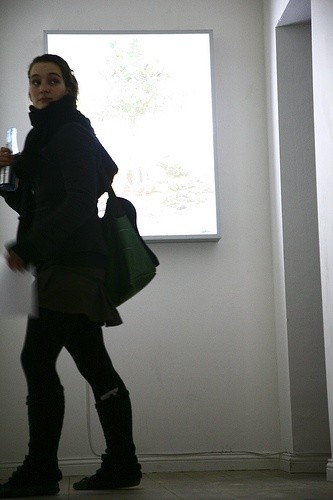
[0, 128, 22, 190]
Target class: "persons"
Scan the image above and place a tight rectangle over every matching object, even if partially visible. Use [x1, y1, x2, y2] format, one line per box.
[0, 53, 144, 498]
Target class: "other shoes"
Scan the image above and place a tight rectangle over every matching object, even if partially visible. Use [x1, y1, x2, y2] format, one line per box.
[0, 452, 64, 499]
[71, 449, 141, 492]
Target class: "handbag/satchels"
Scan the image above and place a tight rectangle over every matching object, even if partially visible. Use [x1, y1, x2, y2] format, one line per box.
[102, 197, 160, 310]
